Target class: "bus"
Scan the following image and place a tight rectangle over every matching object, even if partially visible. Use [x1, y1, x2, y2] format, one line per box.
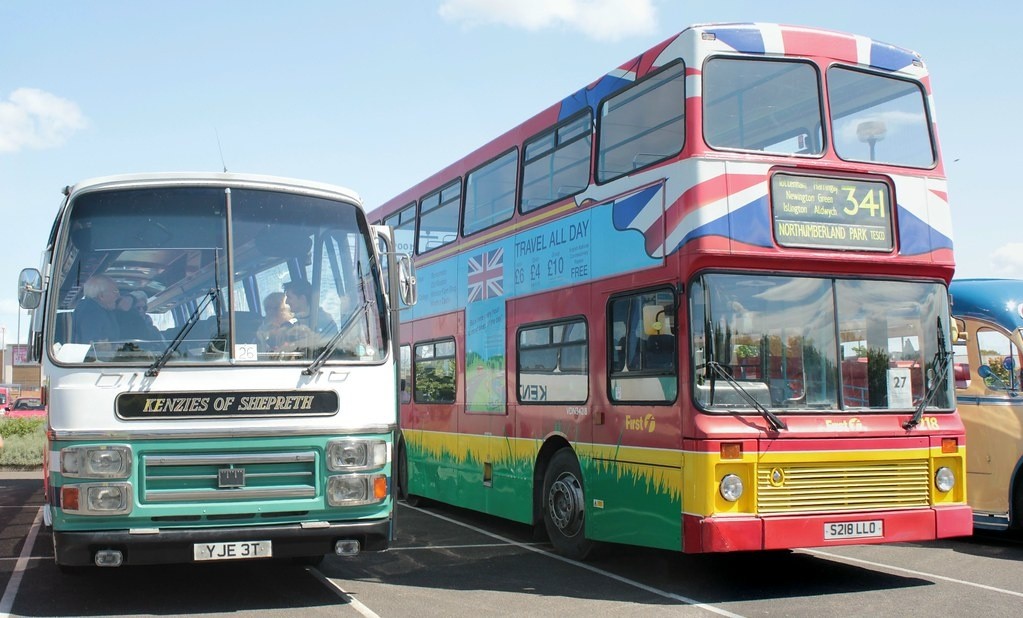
[726, 275, 1022, 549]
[18, 171, 421, 572]
[367, 21, 976, 565]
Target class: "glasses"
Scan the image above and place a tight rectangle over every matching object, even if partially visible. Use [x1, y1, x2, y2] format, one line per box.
[136, 306, 147, 311]
[100, 288, 120, 295]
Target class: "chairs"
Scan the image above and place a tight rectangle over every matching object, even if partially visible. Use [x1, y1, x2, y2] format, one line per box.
[424, 154, 673, 254]
[618, 334, 676, 376]
[161, 311, 265, 354]
[19, 402, 27, 410]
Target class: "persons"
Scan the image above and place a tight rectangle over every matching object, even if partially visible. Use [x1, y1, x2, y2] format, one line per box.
[256, 292, 296, 359]
[72, 277, 137, 353]
[128, 290, 165, 349]
[281, 280, 341, 360]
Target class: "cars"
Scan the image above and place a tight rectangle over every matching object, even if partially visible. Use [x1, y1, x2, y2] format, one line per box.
[4, 395, 48, 420]
[0, 387, 15, 415]
